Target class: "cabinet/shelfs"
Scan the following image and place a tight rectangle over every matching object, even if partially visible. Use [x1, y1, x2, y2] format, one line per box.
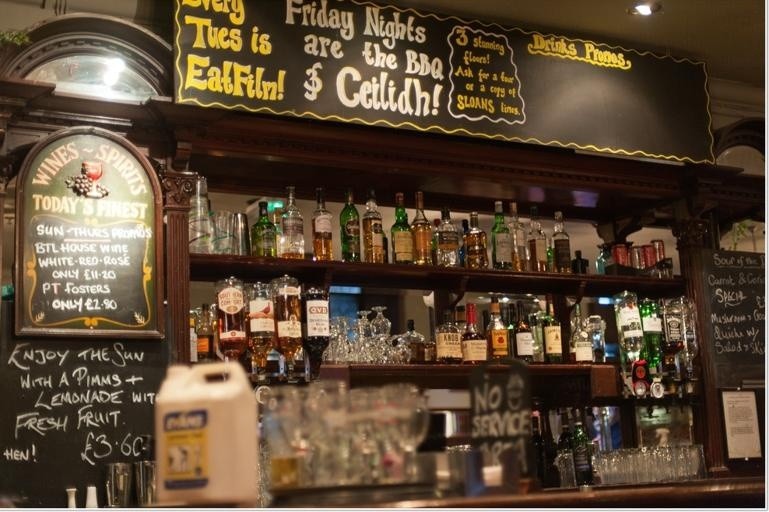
[166, 145, 730, 480]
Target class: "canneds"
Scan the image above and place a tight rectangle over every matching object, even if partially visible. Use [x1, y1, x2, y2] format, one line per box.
[642, 244, 657, 270]
[612, 240, 628, 272]
[651, 240, 665, 262]
[629, 244, 646, 276]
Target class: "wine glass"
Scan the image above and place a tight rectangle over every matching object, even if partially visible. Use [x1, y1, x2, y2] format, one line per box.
[259, 379, 431, 490]
[322, 306, 411, 365]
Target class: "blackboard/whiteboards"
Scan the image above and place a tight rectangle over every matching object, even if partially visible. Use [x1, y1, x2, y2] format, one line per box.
[0, 300, 171, 508]
[471, 357, 533, 479]
[172, 0, 716, 167]
[15, 126, 166, 340]
[703, 248, 765, 386]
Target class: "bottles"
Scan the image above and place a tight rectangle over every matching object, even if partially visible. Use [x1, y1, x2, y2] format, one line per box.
[512, 300, 534, 365]
[311, 186, 334, 260]
[664, 301, 683, 377]
[558, 425, 572, 450]
[490, 303, 510, 358]
[613, 291, 643, 363]
[462, 303, 489, 364]
[410, 190, 434, 265]
[639, 296, 663, 375]
[215, 276, 250, 361]
[570, 305, 586, 346]
[341, 186, 361, 262]
[251, 202, 278, 257]
[543, 304, 564, 362]
[491, 200, 514, 269]
[531, 416, 546, 480]
[551, 210, 570, 273]
[572, 421, 593, 486]
[434, 308, 463, 363]
[529, 205, 547, 272]
[466, 209, 489, 269]
[508, 202, 528, 272]
[250, 281, 277, 386]
[683, 297, 698, 378]
[436, 207, 461, 266]
[303, 287, 330, 380]
[280, 186, 305, 258]
[460, 219, 470, 266]
[391, 192, 414, 265]
[362, 189, 383, 263]
[406, 319, 426, 363]
[273, 274, 302, 377]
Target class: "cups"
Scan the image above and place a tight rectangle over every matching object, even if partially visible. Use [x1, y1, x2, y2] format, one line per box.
[558, 448, 578, 489]
[593, 444, 708, 484]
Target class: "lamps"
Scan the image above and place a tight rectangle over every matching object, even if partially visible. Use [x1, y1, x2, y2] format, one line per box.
[625, 0, 662, 17]
